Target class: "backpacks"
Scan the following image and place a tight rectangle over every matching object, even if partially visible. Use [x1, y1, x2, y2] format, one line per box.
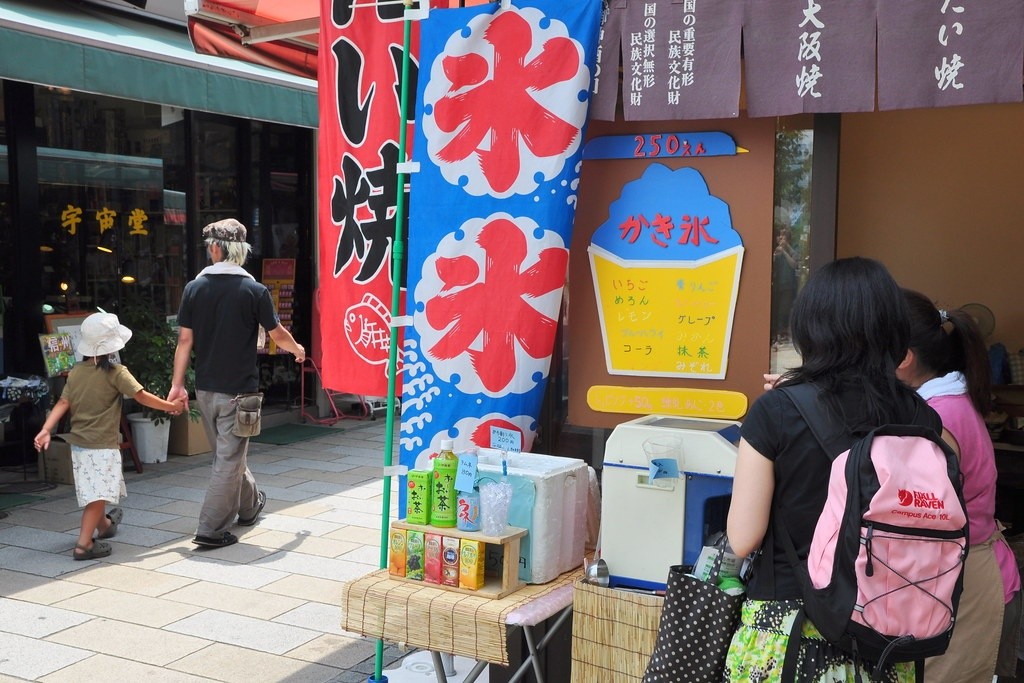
[776, 379, 970, 682]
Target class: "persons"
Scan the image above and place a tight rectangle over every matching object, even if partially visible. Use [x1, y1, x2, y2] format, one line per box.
[764, 288, 1024, 683]
[724, 257, 943, 683]
[165, 219, 305, 547]
[34, 309, 186, 561]
[772, 227, 798, 347]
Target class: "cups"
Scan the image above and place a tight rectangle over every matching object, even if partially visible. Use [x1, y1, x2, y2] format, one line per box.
[480, 481, 506, 537]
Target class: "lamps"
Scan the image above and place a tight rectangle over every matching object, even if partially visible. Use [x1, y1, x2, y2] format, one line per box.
[120, 259, 136, 284]
[37, 219, 64, 252]
[60, 278, 72, 290]
[96, 228, 119, 254]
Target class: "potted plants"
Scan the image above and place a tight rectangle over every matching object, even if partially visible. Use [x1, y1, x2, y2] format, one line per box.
[118, 300, 201, 465]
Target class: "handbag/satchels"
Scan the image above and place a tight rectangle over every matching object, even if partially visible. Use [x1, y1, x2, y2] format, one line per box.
[229, 392, 264, 438]
[642, 529, 762, 683]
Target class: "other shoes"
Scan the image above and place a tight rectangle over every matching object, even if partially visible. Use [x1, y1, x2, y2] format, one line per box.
[237, 490, 266, 527]
[192, 531, 237, 547]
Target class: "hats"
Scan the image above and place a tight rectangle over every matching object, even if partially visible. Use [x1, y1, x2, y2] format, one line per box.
[202, 218, 247, 242]
[77, 305, 133, 366]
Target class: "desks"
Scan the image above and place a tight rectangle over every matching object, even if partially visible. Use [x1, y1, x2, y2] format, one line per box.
[342, 548, 597, 683]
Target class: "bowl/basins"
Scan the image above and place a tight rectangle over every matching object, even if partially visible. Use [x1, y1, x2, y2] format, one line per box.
[585, 559, 609, 588]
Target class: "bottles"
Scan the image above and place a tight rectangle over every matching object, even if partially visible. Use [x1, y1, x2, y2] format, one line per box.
[457, 488, 480, 531]
[430, 440, 457, 528]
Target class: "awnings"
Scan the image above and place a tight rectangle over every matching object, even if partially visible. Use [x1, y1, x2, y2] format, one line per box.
[163, 189, 186, 213]
[0, 0, 319, 129]
[0, 145, 163, 192]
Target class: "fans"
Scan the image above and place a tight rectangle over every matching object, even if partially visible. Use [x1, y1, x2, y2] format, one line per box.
[960, 303, 994, 340]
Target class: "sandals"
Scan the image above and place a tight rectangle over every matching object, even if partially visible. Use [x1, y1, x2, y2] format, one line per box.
[98, 508, 123, 539]
[73, 538, 112, 561]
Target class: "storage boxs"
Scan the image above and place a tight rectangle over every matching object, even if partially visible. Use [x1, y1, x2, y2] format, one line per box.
[455, 446, 588, 586]
[389, 528, 485, 591]
[37, 434, 76, 485]
[170, 400, 213, 456]
